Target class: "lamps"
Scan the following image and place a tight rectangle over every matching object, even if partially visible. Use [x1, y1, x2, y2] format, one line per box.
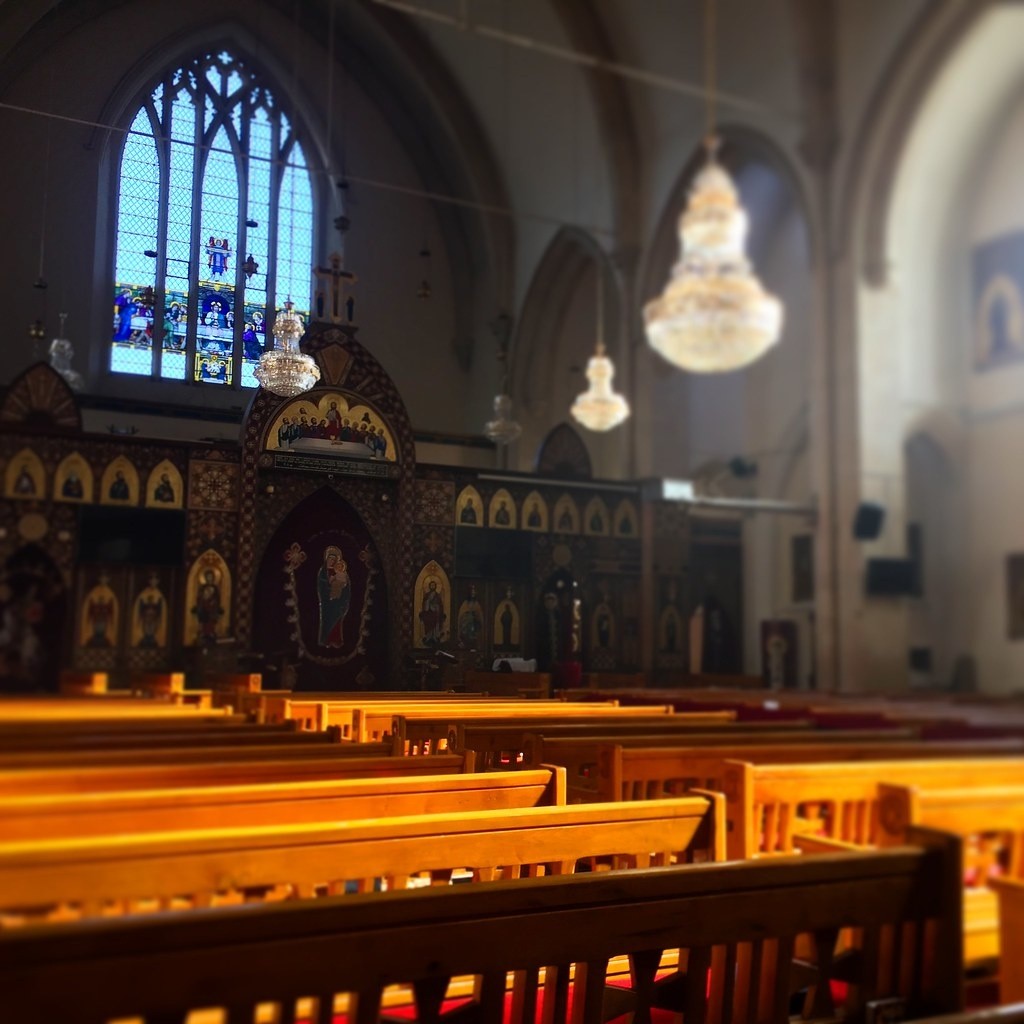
[639, 0, 786, 375]
[251, 0, 323, 395]
[567, 230, 632, 432]
[49, 312, 84, 393]
[484, 394, 523, 446]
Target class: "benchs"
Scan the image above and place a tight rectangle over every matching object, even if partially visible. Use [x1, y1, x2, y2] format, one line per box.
[0, 669, 1024, 1024]
